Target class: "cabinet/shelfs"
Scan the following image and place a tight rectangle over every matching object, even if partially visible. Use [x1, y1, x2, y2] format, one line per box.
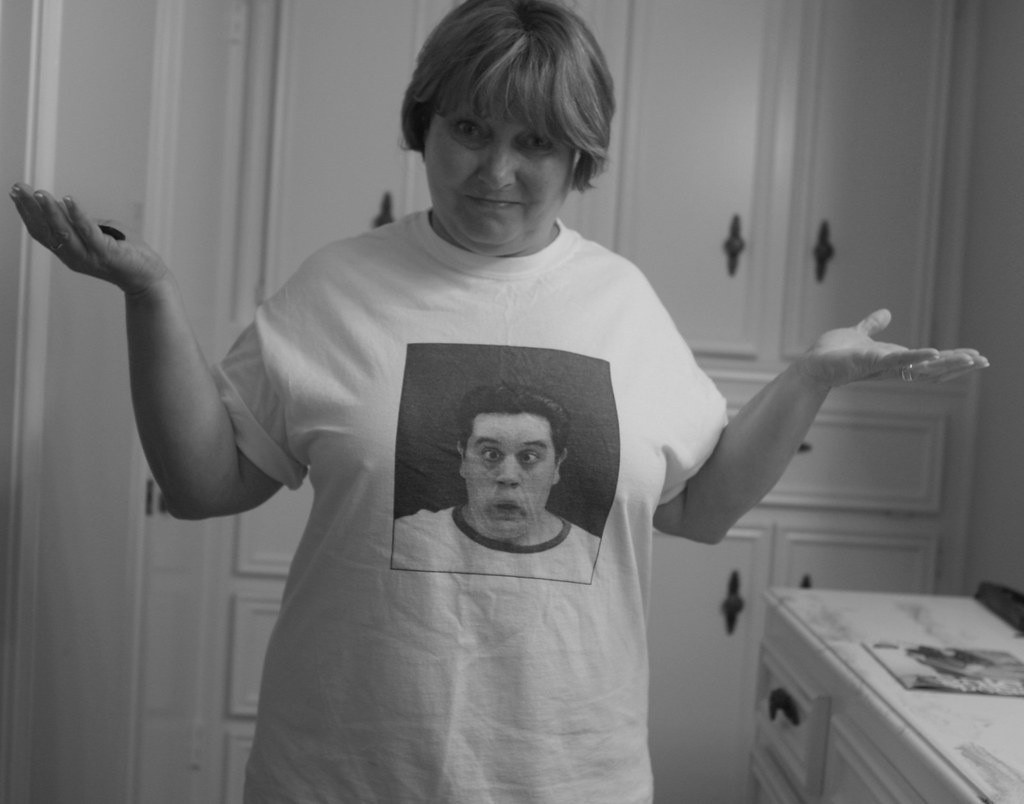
[0, 2, 1023, 804]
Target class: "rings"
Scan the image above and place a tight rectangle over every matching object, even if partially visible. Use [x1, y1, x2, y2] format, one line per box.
[902, 364, 912, 381]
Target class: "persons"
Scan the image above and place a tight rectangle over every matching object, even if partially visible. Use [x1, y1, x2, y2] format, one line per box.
[390, 386, 602, 584]
[8, 0, 989, 804]
